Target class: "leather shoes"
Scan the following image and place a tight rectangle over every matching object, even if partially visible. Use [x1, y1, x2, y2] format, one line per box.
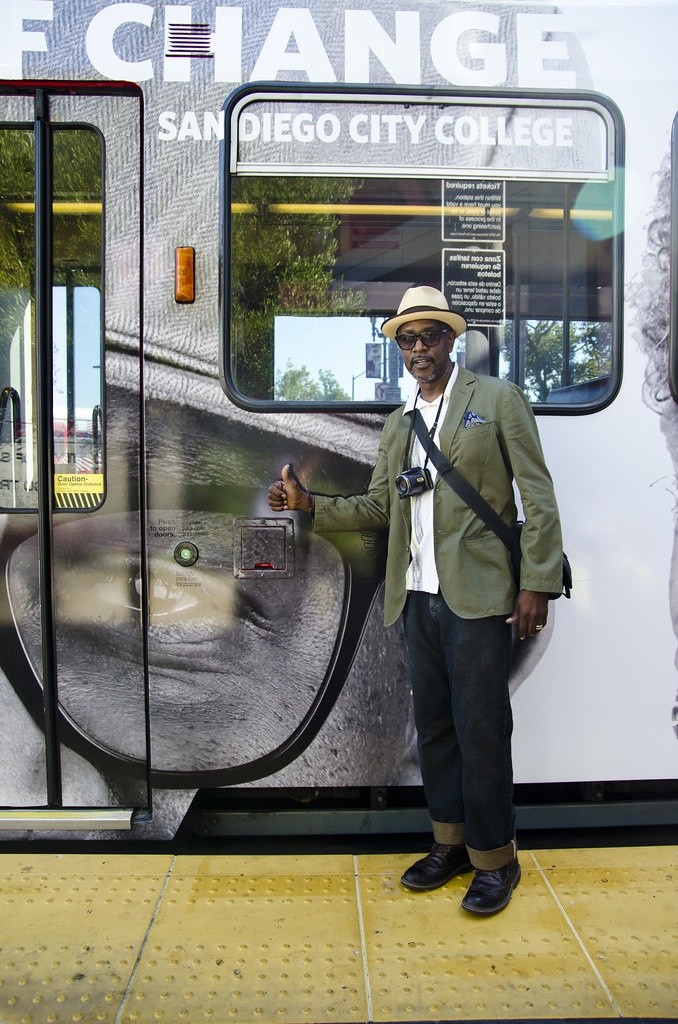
[460, 856, 521, 914]
[400, 842, 476, 891]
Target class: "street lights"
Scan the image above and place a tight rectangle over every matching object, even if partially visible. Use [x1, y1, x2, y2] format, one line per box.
[370, 317, 388, 383]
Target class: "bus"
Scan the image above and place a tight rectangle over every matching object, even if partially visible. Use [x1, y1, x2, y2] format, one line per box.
[0, 0, 676, 857]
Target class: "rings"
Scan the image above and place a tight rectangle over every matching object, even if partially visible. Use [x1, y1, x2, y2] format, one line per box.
[536, 625, 542, 629]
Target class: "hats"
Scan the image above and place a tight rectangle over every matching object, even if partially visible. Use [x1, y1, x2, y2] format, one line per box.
[380, 286, 467, 338]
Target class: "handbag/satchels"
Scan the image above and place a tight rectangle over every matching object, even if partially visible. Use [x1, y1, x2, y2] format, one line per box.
[512, 520, 572, 601]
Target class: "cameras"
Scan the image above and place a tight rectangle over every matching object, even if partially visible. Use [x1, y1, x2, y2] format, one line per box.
[395, 466, 434, 500]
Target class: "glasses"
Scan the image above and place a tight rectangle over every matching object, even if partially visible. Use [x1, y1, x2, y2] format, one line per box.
[394, 328, 450, 350]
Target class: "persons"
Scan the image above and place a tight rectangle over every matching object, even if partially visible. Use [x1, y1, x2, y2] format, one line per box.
[269, 286, 564, 915]
